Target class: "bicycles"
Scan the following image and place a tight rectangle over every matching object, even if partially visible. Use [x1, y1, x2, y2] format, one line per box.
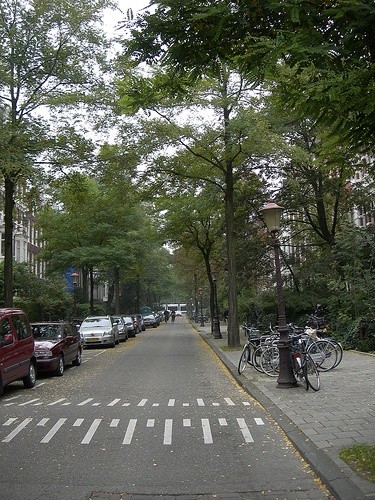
[236, 310, 344, 391]
[172, 315, 176, 323]
[164, 314, 168, 323]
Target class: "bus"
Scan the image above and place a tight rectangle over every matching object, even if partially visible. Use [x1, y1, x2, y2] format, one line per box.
[162, 303, 196, 316]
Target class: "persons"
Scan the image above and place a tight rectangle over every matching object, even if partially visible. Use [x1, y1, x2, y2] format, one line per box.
[164, 309, 169, 321]
[170, 310, 176, 321]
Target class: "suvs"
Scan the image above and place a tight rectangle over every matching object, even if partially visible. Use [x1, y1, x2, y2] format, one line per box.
[30, 321, 84, 377]
[0, 308, 40, 396]
[75, 315, 120, 349]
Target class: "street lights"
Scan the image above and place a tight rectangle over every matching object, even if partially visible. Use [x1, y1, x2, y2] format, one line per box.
[212, 278, 223, 339]
[257, 203, 300, 390]
[198, 288, 205, 327]
[70, 272, 79, 319]
[115, 264, 121, 316]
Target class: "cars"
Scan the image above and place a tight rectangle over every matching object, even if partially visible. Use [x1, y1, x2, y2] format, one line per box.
[122, 316, 136, 337]
[128, 309, 166, 334]
[112, 316, 128, 343]
[175, 311, 181, 316]
